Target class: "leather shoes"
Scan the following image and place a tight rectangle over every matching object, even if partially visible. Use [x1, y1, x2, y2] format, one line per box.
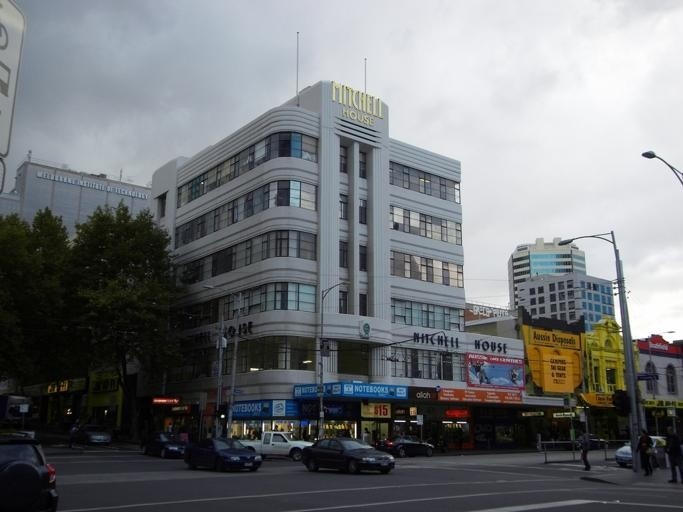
[668, 479, 677, 483]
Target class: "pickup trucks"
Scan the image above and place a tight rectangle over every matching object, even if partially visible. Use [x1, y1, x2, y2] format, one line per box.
[237, 430, 313, 461]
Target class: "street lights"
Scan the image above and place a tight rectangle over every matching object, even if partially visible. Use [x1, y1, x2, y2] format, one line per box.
[202, 285, 242, 439]
[557, 230, 642, 473]
[316, 279, 350, 440]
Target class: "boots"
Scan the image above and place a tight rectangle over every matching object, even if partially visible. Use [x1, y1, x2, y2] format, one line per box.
[644, 469, 652, 476]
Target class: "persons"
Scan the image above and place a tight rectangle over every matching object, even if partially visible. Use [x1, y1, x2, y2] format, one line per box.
[230, 424, 351, 441]
[663, 426, 682, 484]
[399, 419, 561, 453]
[621, 424, 630, 440]
[136, 415, 200, 456]
[578, 427, 590, 471]
[636, 427, 653, 477]
[511, 366, 522, 385]
[478, 368, 489, 384]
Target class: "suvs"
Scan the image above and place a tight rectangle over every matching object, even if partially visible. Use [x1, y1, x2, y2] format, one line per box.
[0, 432, 58, 512]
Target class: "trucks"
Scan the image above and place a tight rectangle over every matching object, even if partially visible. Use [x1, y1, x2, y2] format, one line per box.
[1, 393, 41, 427]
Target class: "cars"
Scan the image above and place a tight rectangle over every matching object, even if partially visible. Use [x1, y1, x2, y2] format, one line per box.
[614, 435, 667, 467]
[377, 434, 434, 457]
[138, 434, 186, 459]
[303, 438, 394, 474]
[76, 425, 111, 445]
[185, 439, 262, 472]
